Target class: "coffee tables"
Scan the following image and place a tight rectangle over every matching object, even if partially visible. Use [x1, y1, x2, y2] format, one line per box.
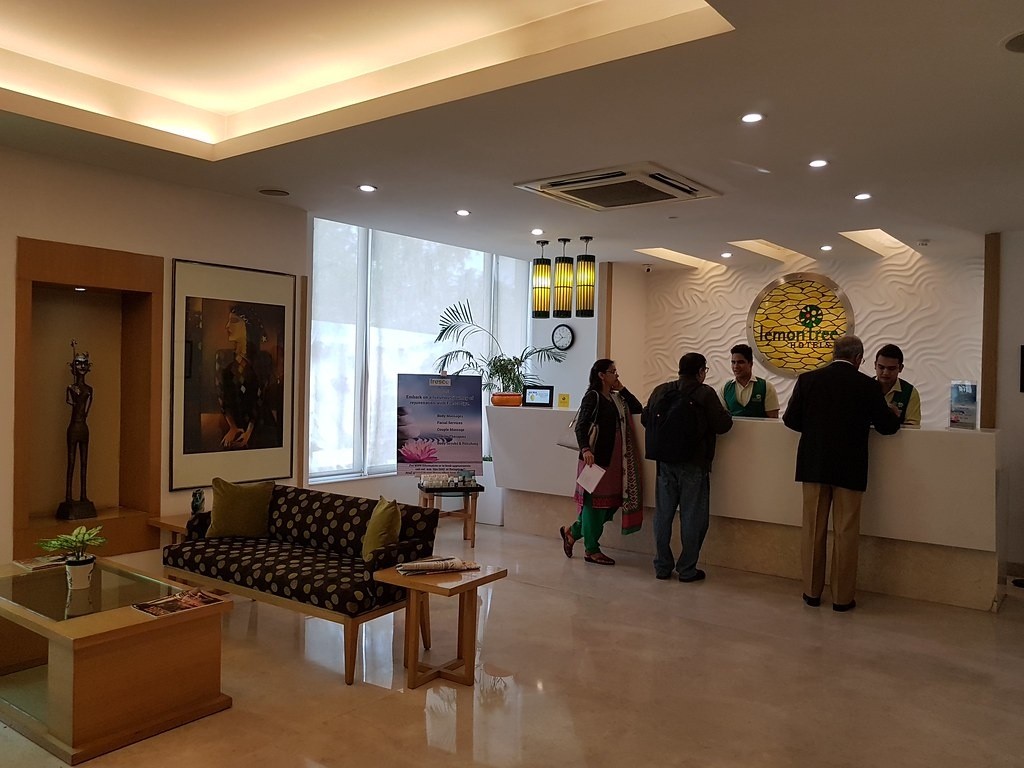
[0, 564, 234, 765]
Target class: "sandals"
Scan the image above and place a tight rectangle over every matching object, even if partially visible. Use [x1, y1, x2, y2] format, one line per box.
[585, 552, 615, 565]
[561, 525, 574, 558]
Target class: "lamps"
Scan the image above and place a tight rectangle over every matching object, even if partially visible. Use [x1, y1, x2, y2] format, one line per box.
[551, 237, 574, 318]
[575, 235, 596, 318]
[531, 238, 550, 320]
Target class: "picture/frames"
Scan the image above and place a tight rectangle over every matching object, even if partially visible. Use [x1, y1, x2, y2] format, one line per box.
[168, 258, 297, 494]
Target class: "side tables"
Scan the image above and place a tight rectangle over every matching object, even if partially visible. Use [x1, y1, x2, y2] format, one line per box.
[372, 557, 506, 693]
[149, 510, 207, 588]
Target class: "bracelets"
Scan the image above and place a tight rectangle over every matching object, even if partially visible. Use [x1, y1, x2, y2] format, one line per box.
[582, 449, 590, 453]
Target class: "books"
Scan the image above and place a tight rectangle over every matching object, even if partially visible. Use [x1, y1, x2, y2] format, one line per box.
[131, 588, 224, 618]
[13, 554, 68, 571]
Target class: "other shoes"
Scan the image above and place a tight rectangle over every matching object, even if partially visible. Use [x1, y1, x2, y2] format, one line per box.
[803, 593, 820, 606]
[680, 569, 705, 582]
[833, 600, 855, 611]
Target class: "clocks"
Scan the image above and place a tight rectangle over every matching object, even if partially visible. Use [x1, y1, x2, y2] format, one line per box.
[552, 325, 574, 351]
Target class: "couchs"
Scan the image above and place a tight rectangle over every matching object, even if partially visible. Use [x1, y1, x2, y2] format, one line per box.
[154, 480, 448, 685]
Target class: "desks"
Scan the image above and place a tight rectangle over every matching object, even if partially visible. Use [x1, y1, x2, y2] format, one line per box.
[418, 481, 485, 546]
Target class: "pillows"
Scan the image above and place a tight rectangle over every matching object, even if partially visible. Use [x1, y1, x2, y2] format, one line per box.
[207, 478, 276, 537]
[361, 495, 400, 561]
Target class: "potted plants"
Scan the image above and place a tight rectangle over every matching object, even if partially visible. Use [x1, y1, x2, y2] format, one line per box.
[490, 356, 527, 406]
[434, 298, 567, 528]
[40, 523, 106, 589]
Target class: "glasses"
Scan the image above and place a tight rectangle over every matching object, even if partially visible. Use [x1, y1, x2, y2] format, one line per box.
[604, 370, 617, 375]
[856, 353, 865, 365]
[702, 367, 709, 373]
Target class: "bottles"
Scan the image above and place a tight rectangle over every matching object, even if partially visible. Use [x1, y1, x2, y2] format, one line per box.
[423, 474, 476, 488]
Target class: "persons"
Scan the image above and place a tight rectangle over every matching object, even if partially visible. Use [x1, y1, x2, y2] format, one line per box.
[782, 336, 901, 612]
[561, 359, 643, 565]
[718, 344, 781, 418]
[66, 351, 93, 501]
[640, 353, 733, 582]
[869, 345, 921, 425]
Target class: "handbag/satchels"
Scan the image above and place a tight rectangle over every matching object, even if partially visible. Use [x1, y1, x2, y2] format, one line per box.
[557, 390, 600, 453]
